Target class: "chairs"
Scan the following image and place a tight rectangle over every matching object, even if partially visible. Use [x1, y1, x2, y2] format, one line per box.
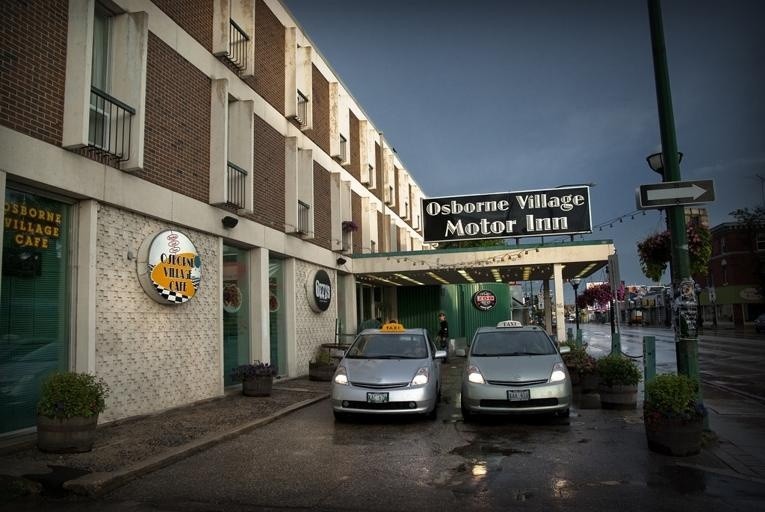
[478, 334, 544, 354]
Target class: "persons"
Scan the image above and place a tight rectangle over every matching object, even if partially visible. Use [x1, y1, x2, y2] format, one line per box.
[435, 310, 450, 362]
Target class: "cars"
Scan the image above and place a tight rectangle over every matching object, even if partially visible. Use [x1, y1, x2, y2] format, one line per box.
[330, 323, 448, 425]
[461, 320, 573, 426]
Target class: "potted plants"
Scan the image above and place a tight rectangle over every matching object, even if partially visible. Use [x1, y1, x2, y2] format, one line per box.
[555, 341, 643, 409]
[309, 352, 337, 382]
[33, 370, 110, 454]
[231, 363, 279, 397]
[643, 372, 705, 457]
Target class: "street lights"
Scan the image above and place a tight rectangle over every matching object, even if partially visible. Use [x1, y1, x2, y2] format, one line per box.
[694, 288, 702, 329]
[569, 278, 581, 330]
[606, 264, 615, 334]
[646, 151, 683, 284]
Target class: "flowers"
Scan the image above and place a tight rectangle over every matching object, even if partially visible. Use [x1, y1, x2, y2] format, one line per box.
[574, 283, 624, 308]
[636, 214, 712, 282]
